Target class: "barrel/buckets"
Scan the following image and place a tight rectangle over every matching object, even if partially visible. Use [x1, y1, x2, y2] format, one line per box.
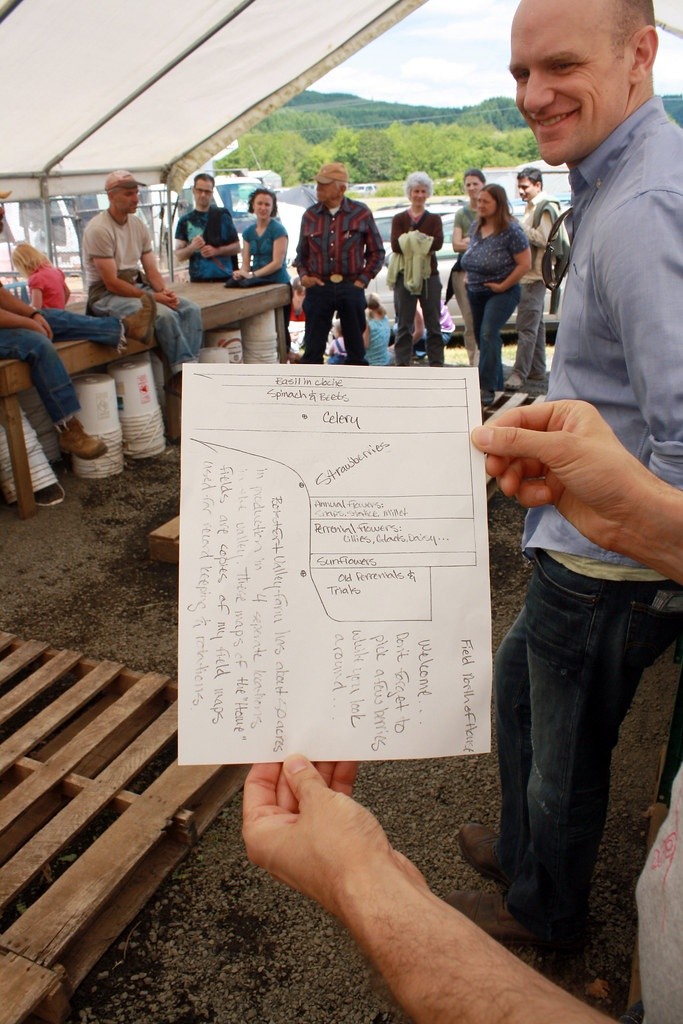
[198, 311, 280, 368]
[0, 360, 166, 504]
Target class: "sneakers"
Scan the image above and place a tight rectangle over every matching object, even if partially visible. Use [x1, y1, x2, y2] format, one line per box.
[445, 890, 544, 946]
[457, 823, 512, 888]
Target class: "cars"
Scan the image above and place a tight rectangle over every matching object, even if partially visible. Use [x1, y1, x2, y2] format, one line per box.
[351, 183, 378, 195]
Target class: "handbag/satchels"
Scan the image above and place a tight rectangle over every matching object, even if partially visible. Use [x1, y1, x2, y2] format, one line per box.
[384, 252, 395, 268]
[291, 272, 310, 321]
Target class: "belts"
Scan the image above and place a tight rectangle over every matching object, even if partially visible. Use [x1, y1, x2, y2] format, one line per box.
[321, 274, 357, 284]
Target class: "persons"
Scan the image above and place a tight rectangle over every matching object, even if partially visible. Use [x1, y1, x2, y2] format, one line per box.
[442, 0, 683, 951]
[292, 162, 385, 365]
[174, 173, 240, 282]
[80, 170, 203, 377]
[503, 167, 569, 392]
[391, 172, 445, 367]
[460, 184, 532, 404]
[243, 399, 683, 1024]
[288, 277, 456, 367]
[0, 190, 156, 460]
[12, 243, 71, 310]
[451, 168, 486, 367]
[231, 188, 293, 350]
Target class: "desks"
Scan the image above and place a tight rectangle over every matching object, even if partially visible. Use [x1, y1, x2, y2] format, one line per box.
[0, 277, 293, 518]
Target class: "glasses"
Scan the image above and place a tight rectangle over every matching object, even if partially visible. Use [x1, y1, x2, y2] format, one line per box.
[541, 207, 576, 292]
[195, 187, 212, 194]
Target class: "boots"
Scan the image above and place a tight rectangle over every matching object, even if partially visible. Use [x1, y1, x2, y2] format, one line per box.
[122, 293, 156, 345]
[59, 418, 107, 461]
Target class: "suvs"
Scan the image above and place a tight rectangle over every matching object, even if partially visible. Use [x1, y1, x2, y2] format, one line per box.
[372, 196, 572, 338]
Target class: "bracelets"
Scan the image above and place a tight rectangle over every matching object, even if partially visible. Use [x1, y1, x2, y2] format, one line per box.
[252, 271, 256, 278]
[30, 311, 39, 318]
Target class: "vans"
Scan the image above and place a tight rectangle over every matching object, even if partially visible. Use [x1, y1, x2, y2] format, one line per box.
[2, 177, 263, 274]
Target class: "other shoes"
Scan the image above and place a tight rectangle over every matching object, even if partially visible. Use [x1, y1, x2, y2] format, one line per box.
[480, 390, 495, 404]
[162, 372, 182, 396]
[529, 372, 545, 380]
[504, 375, 524, 389]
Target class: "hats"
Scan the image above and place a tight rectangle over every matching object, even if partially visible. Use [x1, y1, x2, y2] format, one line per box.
[105, 170, 145, 188]
[314, 163, 347, 184]
